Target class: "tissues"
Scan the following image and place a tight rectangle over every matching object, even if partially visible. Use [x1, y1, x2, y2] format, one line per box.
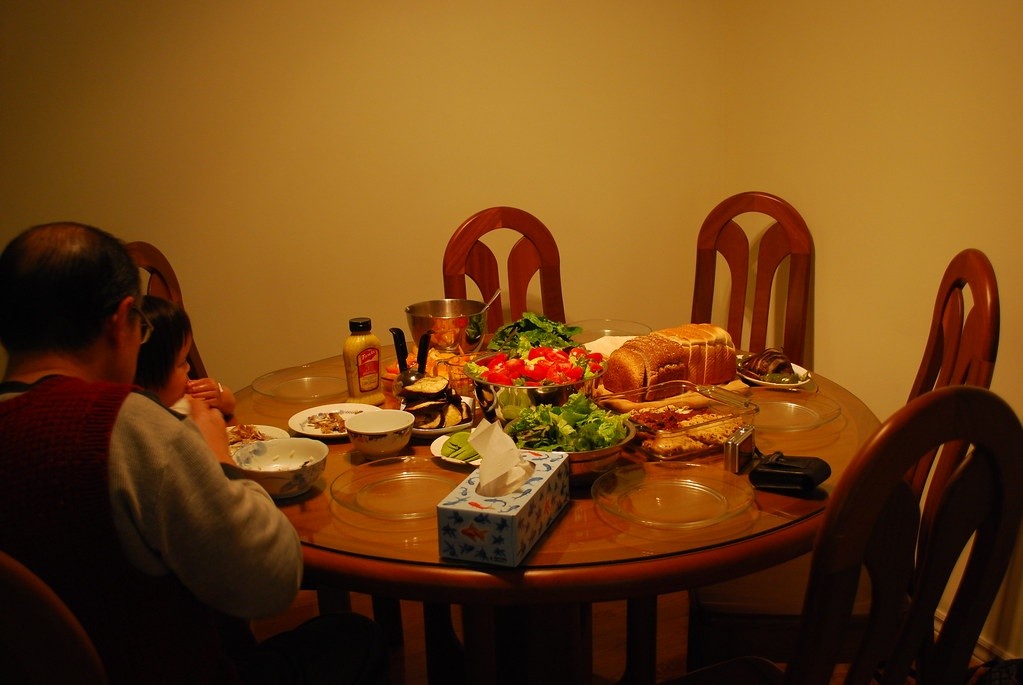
[437, 418, 570, 569]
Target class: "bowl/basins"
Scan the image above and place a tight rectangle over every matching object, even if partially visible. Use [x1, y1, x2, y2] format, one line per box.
[593, 380, 760, 460]
[345, 410, 415, 460]
[232, 438, 329, 498]
[405, 299, 488, 353]
[463, 351, 608, 429]
[504, 411, 636, 483]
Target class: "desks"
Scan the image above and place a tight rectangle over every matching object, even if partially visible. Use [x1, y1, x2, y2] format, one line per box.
[226, 332, 880, 685]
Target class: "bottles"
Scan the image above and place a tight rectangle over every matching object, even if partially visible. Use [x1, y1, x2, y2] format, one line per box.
[343, 318, 386, 405]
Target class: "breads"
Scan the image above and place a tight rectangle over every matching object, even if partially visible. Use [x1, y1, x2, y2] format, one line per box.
[601, 322, 736, 404]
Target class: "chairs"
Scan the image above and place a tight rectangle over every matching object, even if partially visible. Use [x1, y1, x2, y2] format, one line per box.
[0, 196, 1023, 685]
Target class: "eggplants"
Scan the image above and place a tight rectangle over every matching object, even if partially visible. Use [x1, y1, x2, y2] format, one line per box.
[397, 376, 472, 429]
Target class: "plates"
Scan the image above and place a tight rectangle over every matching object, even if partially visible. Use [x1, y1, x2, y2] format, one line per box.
[225, 425, 290, 466]
[591, 461, 754, 530]
[430, 427, 483, 466]
[736, 355, 811, 388]
[731, 388, 842, 432]
[564, 319, 652, 355]
[288, 403, 382, 439]
[252, 366, 349, 401]
[400, 396, 475, 438]
[331, 456, 477, 522]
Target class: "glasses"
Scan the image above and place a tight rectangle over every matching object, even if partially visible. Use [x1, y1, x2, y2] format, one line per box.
[130, 305, 153, 344]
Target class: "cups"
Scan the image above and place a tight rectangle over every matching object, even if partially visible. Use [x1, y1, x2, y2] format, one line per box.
[433, 355, 485, 409]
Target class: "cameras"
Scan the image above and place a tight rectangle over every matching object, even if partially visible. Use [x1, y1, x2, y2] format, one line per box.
[724, 424, 756, 474]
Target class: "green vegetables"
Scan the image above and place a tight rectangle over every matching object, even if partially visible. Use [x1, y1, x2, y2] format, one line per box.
[466, 314, 485, 340]
[508, 392, 636, 454]
[487, 312, 584, 355]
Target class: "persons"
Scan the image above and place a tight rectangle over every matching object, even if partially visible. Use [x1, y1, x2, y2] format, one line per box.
[0, 221, 375, 685]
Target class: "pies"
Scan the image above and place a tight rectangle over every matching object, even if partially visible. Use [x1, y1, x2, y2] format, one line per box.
[641, 412, 745, 455]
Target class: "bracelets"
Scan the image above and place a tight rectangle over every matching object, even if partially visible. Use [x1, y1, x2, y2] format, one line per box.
[218, 383, 223, 392]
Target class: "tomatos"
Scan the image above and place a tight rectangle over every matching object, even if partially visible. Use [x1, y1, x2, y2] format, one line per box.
[480, 345, 603, 389]
